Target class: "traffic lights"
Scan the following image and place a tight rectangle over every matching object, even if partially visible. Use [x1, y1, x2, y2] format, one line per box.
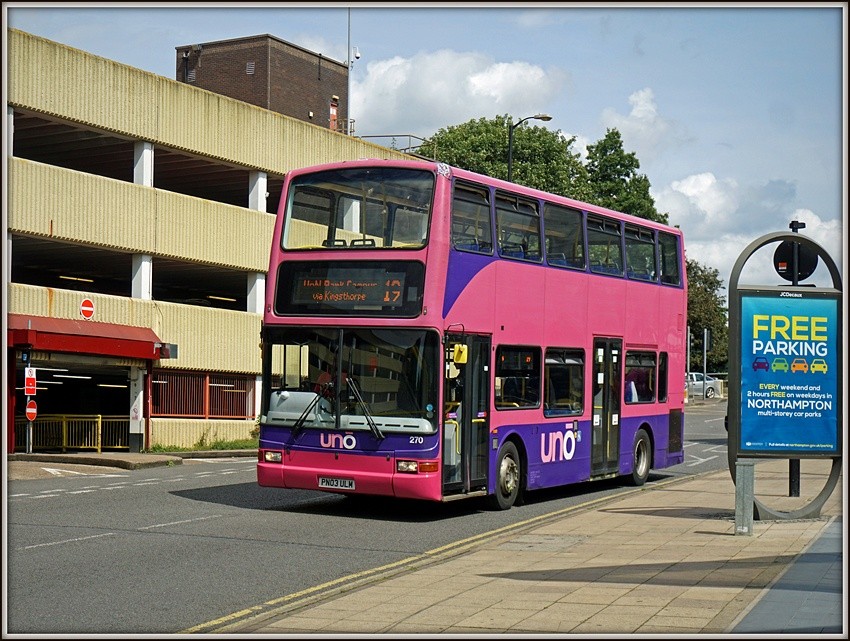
[706, 328, 714, 351]
[690, 334, 694, 352]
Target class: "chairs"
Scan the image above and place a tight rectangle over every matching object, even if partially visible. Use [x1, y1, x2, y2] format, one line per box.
[321, 239, 676, 285]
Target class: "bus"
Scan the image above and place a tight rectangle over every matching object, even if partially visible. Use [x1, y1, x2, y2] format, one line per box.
[256, 160, 689, 511]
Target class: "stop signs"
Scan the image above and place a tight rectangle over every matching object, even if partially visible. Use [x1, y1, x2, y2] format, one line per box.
[26, 401, 37, 421]
[81, 299, 95, 319]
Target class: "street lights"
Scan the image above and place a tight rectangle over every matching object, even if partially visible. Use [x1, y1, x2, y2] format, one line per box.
[506, 114, 552, 179]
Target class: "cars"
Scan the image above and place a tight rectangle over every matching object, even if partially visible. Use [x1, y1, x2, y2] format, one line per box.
[685, 373, 716, 399]
